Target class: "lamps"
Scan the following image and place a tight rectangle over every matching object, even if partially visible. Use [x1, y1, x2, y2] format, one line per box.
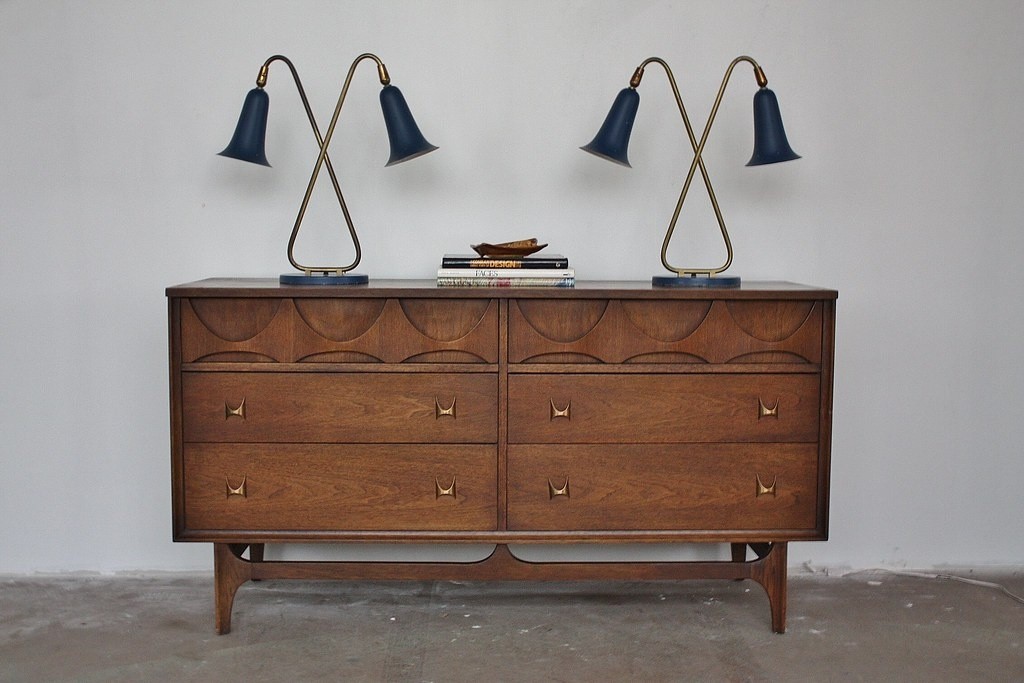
[578, 52, 802, 288]
[217, 52, 438, 286]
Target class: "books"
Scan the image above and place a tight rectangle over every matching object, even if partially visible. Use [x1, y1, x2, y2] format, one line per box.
[438, 251, 575, 288]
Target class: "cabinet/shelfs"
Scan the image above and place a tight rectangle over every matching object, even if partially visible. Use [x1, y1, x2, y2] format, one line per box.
[165, 277, 839, 635]
